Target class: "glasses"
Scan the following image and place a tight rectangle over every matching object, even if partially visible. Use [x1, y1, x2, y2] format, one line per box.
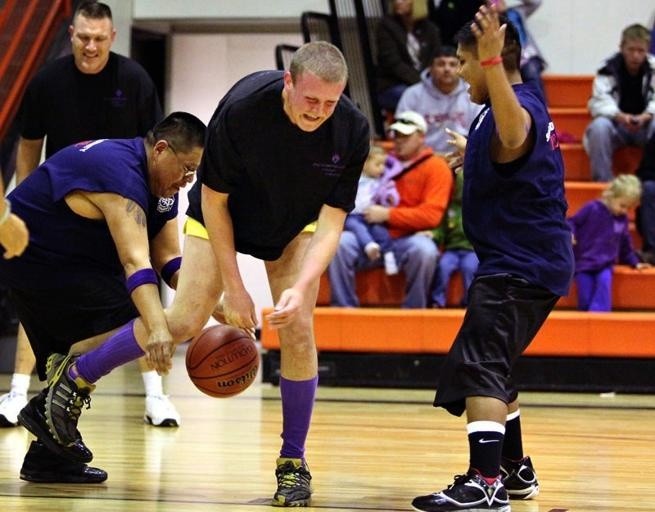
[165, 145, 196, 177]
[391, 118, 417, 126]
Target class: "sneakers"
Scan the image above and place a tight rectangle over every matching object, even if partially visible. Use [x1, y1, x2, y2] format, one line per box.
[144, 394, 181, 428]
[500, 455, 542, 501]
[44, 353, 97, 447]
[17, 397, 92, 463]
[0, 392, 30, 428]
[272, 457, 313, 508]
[411, 469, 513, 512]
[20, 440, 108, 485]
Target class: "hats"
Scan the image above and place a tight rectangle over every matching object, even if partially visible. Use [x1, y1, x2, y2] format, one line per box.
[387, 111, 428, 136]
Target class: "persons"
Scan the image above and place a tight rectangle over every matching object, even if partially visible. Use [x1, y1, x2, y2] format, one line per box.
[329, 0, 541, 306]
[582, 24, 655, 183]
[0, 111, 256, 484]
[0, 2, 179, 427]
[412, 5, 575, 512]
[634, 130, 655, 263]
[565, 174, 647, 310]
[41, 40, 371, 506]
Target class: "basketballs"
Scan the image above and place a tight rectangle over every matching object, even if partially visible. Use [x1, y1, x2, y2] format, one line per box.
[186, 324, 259, 398]
